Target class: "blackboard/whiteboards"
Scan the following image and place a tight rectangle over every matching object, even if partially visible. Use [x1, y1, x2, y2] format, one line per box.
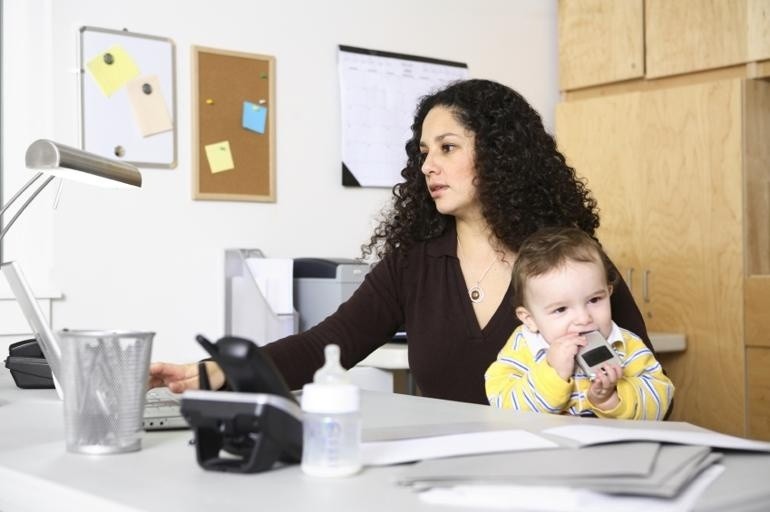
[79, 26, 177, 170]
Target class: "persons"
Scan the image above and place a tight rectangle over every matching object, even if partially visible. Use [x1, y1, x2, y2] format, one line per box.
[482, 223, 676, 419]
[144, 78, 676, 421]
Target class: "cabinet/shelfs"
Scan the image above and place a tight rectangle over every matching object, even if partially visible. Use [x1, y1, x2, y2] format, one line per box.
[552, 77, 767, 444]
[556, 0, 768, 93]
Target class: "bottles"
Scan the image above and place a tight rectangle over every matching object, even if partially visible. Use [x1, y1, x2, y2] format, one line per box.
[301, 342, 365, 475]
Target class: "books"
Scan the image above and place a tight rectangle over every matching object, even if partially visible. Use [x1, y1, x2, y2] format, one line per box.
[361, 407, 770, 510]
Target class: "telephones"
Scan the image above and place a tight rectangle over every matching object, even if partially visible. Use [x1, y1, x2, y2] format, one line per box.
[181, 335, 302, 470]
[4, 339, 55, 389]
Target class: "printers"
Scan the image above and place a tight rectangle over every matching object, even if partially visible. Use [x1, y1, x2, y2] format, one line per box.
[293, 257, 407, 343]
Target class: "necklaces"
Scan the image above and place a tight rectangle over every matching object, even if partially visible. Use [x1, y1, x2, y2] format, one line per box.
[455, 228, 505, 303]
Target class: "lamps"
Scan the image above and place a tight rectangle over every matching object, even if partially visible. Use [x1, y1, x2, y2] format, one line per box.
[0, 133, 142, 391]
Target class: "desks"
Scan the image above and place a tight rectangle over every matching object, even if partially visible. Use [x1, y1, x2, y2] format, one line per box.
[0, 366, 770, 512]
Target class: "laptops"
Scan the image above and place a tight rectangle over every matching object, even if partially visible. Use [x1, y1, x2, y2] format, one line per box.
[1, 260, 191, 430]
